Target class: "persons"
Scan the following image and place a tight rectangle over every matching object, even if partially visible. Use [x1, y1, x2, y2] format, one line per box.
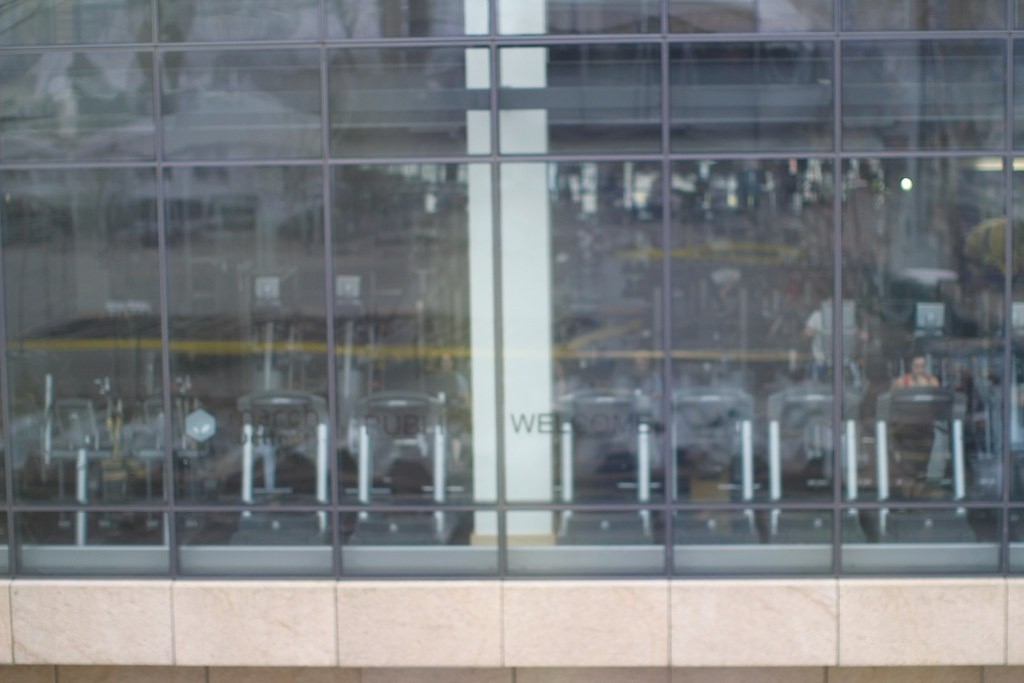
[891, 355, 940, 498]
[802, 308, 869, 385]
